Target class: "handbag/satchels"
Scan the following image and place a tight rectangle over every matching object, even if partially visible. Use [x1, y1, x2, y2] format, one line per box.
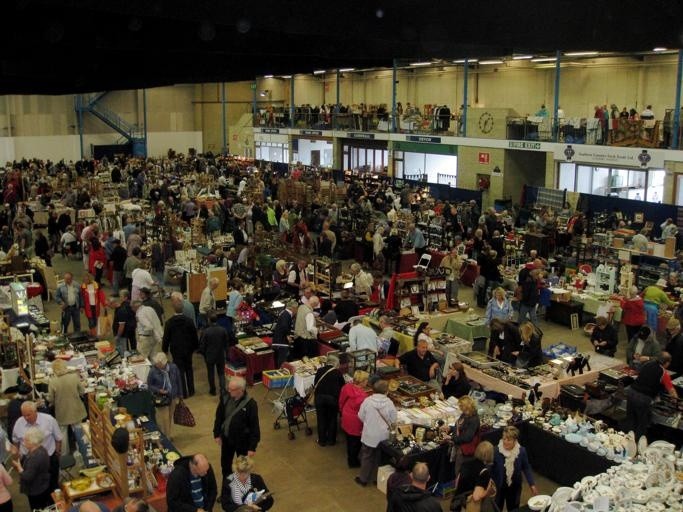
[390, 430, 396, 442]
[306, 392, 315, 407]
[153, 394, 169, 407]
[100, 308, 113, 334]
[47, 249, 55, 258]
[173, 399, 196, 427]
[305, 407, 317, 429]
[459, 430, 482, 455]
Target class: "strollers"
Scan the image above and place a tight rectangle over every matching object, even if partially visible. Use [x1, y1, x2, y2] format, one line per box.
[271, 384, 316, 441]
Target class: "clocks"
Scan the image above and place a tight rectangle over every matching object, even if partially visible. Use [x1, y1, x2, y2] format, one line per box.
[477, 111, 495, 135]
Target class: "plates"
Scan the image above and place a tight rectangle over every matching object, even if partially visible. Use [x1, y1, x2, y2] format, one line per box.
[565, 433, 631, 466]
[527, 464, 682, 512]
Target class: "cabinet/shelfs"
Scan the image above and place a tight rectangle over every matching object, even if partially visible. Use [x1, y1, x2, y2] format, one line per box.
[0, 154, 683, 512]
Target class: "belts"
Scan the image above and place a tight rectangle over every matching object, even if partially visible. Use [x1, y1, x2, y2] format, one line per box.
[139, 334, 151, 336]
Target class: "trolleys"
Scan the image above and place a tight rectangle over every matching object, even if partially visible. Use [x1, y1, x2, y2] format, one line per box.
[583, 312, 613, 338]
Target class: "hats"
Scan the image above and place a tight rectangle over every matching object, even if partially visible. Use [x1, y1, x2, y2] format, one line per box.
[654, 278, 668, 288]
[269, 301, 285, 309]
[637, 327, 650, 340]
[18, 383, 33, 395]
[140, 288, 150, 296]
[112, 239, 121, 244]
[111, 427, 128, 453]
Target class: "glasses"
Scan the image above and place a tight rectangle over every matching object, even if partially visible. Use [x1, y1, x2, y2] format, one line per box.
[226, 387, 239, 392]
[123, 504, 128, 512]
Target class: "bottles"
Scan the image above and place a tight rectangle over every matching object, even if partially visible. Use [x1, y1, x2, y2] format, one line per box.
[433, 429, 436, 437]
[126, 448, 140, 478]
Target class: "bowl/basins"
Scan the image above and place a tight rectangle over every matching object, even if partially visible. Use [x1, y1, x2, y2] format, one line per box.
[69, 476, 92, 490]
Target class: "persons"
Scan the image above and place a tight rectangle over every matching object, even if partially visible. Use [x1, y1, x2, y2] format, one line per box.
[8, 426, 49, 511]
[48, 359, 88, 465]
[220, 454, 274, 511]
[386, 460, 442, 512]
[356, 380, 397, 487]
[264, 100, 655, 145]
[450, 440, 496, 511]
[339, 370, 370, 469]
[0, 464, 13, 510]
[11, 401, 63, 511]
[386, 456, 412, 504]
[1, 148, 682, 440]
[165, 453, 217, 511]
[442, 395, 480, 478]
[313, 356, 345, 447]
[211, 378, 260, 502]
[488, 425, 537, 511]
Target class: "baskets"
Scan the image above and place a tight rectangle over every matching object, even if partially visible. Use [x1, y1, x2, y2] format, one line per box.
[397, 376, 437, 402]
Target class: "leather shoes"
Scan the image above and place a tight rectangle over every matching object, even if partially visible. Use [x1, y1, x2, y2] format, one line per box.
[356, 477, 367, 486]
